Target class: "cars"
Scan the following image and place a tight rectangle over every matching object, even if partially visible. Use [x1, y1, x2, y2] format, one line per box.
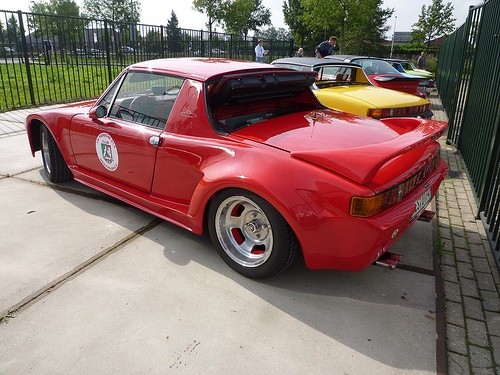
[269, 57, 434, 122]
[121, 46, 137, 53]
[322, 55, 428, 100]
[3, 47, 15, 54]
[382, 58, 436, 88]
[212, 48, 227, 55]
[25, 56, 450, 281]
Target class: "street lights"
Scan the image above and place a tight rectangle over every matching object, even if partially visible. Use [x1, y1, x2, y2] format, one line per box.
[389, 15, 397, 56]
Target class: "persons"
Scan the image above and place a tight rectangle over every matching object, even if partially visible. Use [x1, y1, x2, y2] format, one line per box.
[254, 39, 269, 63]
[315, 36, 338, 59]
[294, 48, 304, 57]
[417, 52, 426, 69]
